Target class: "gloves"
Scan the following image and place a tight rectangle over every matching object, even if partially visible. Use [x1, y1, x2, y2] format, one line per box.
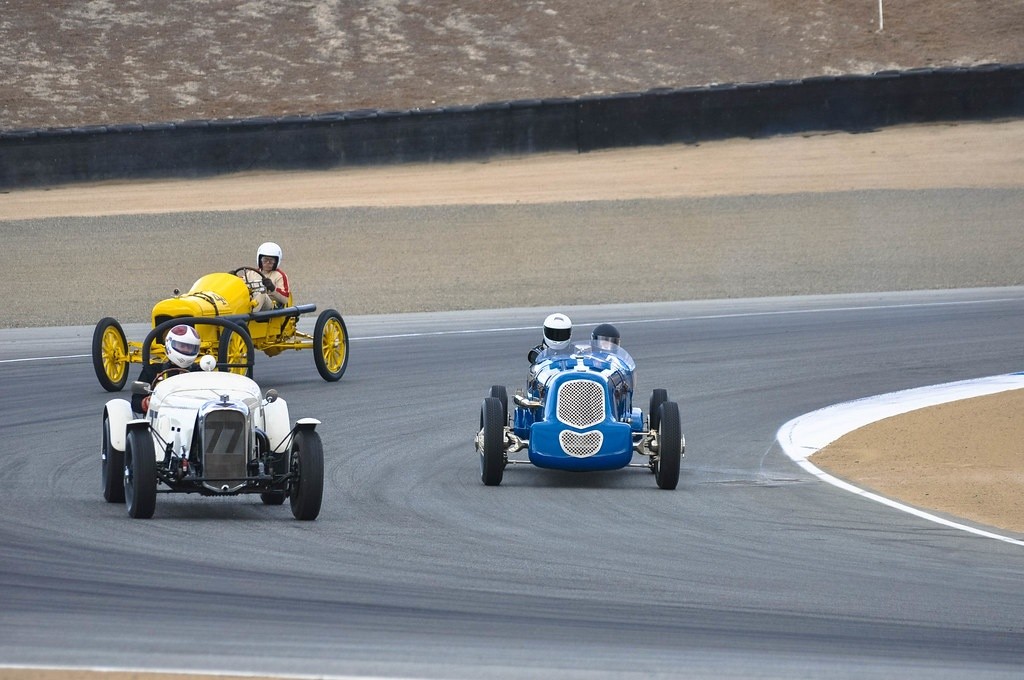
[262, 277, 276, 293]
[229, 269, 236, 275]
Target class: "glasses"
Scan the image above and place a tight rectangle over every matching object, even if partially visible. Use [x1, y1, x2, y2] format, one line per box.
[259, 257, 275, 263]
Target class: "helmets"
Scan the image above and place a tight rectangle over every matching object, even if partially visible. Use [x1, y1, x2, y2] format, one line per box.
[592, 324, 621, 353]
[542, 313, 573, 349]
[257, 242, 283, 269]
[164, 324, 201, 368]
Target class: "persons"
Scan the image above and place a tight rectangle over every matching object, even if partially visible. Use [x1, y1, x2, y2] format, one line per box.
[527, 313, 579, 365]
[131, 324, 204, 414]
[581, 324, 621, 353]
[229, 242, 289, 324]
